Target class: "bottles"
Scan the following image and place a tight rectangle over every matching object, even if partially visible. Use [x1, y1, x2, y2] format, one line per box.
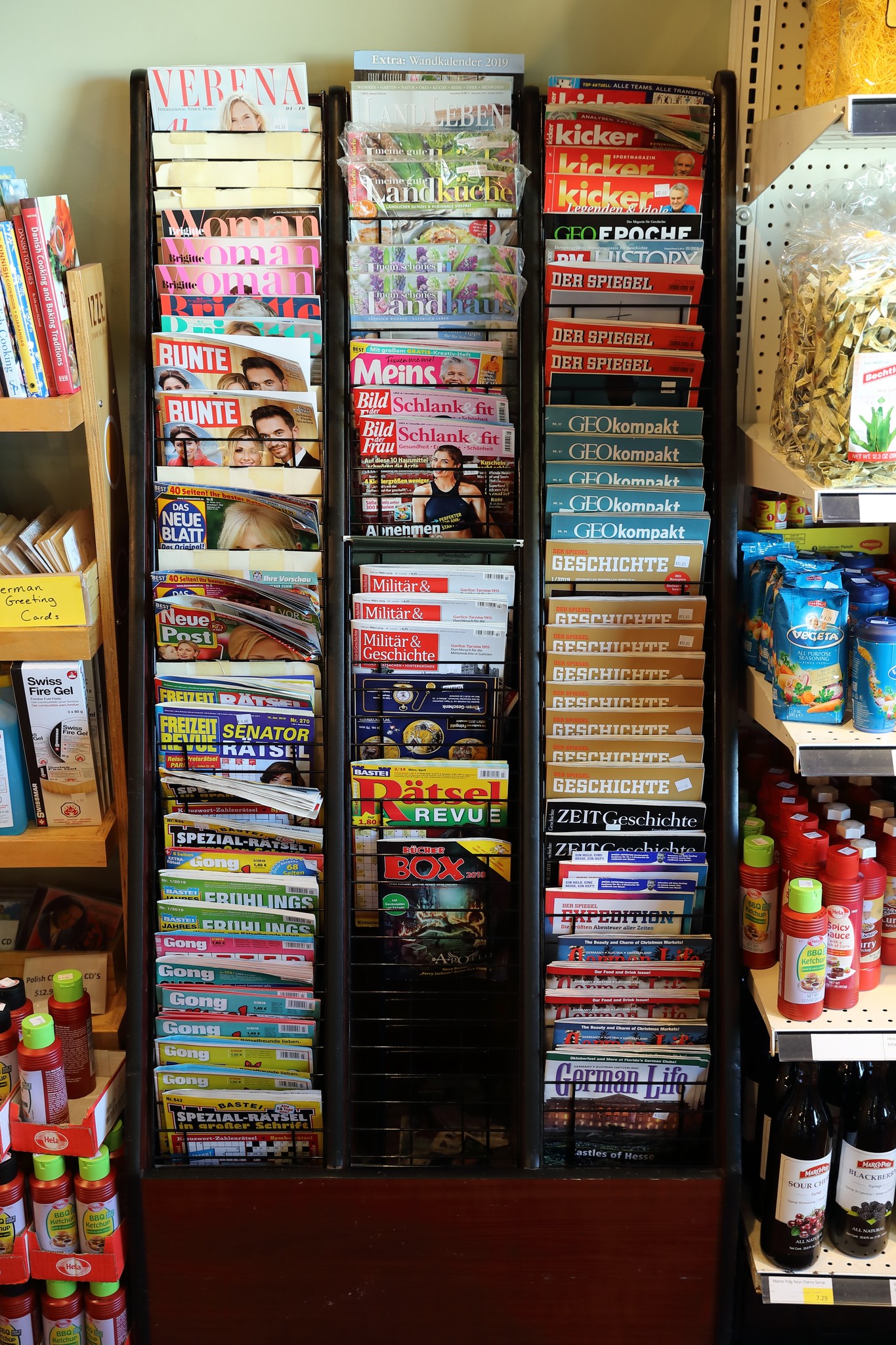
[743, 754, 896, 1020]
[744, 998, 896, 1275]
[0, 970, 129, 1345]
[750, 486, 812, 531]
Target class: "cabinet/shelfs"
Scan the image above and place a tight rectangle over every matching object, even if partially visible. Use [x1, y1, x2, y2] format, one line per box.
[0, 259, 124, 1050]
[745, 93, 896, 1304]
[133, 48, 741, 1191]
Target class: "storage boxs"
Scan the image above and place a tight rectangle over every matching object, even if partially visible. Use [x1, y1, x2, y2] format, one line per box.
[9, 1049, 125, 1156]
[26, 1207, 129, 1282]
[1, 1215, 33, 1285]
[0, 1078, 24, 1164]
[11, 661, 103, 826]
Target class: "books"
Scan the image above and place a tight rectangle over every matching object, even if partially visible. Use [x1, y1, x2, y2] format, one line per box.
[0, 52, 714, 1177]
